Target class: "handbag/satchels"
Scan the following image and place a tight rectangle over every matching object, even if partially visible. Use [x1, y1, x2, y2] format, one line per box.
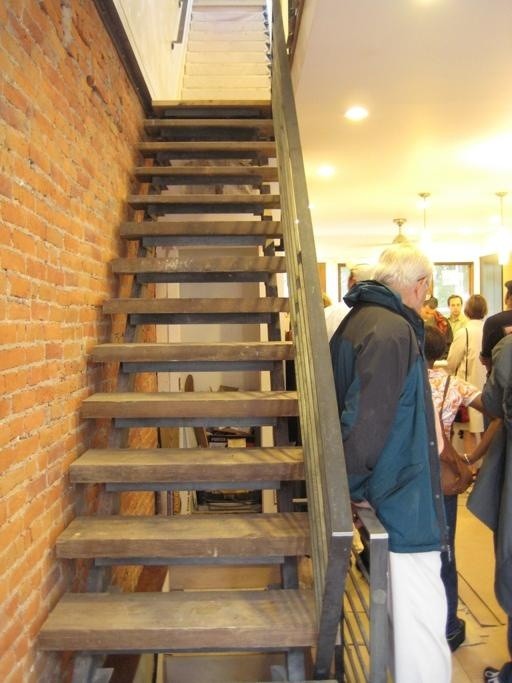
[438, 440, 473, 496]
[453, 404, 470, 423]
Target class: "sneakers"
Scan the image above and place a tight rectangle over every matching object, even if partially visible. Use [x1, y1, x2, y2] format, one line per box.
[483, 666, 502, 683]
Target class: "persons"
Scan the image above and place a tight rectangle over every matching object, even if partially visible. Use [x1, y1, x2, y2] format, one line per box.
[326, 241, 511, 682]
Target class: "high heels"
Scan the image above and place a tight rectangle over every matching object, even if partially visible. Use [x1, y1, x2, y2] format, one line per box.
[446, 618, 465, 652]
